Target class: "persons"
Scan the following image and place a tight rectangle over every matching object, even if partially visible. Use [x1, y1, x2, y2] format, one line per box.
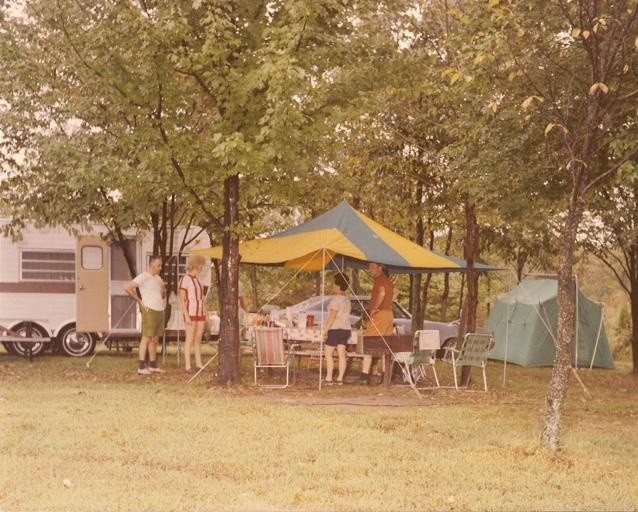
[352, 261, 394, 385]
[125, 255, 168, 376]
[178, 255, 211, 374]
[321, 273, 352, 385]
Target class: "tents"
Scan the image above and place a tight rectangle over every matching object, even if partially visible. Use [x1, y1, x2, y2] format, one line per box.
[482, 273, 616, 368]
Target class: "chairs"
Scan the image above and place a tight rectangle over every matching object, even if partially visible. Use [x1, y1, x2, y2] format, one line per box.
[250, 327, 296, 390]
[388, 329, 496, 399]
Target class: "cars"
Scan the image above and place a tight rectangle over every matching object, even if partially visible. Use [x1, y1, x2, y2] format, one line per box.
[206, 294, 461, 361]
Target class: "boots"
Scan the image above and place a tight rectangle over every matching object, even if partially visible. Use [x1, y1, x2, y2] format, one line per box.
[353, 372, 369, 385]
[380, 372, 385, 384]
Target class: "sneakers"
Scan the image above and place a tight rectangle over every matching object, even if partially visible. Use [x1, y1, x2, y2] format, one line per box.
[322, 379, 343, 386]
[184, 366, 210, 372]
[137, 366, 167, 375]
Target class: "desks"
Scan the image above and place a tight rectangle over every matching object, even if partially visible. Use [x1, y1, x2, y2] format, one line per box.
[282, 320, 404, 384]
